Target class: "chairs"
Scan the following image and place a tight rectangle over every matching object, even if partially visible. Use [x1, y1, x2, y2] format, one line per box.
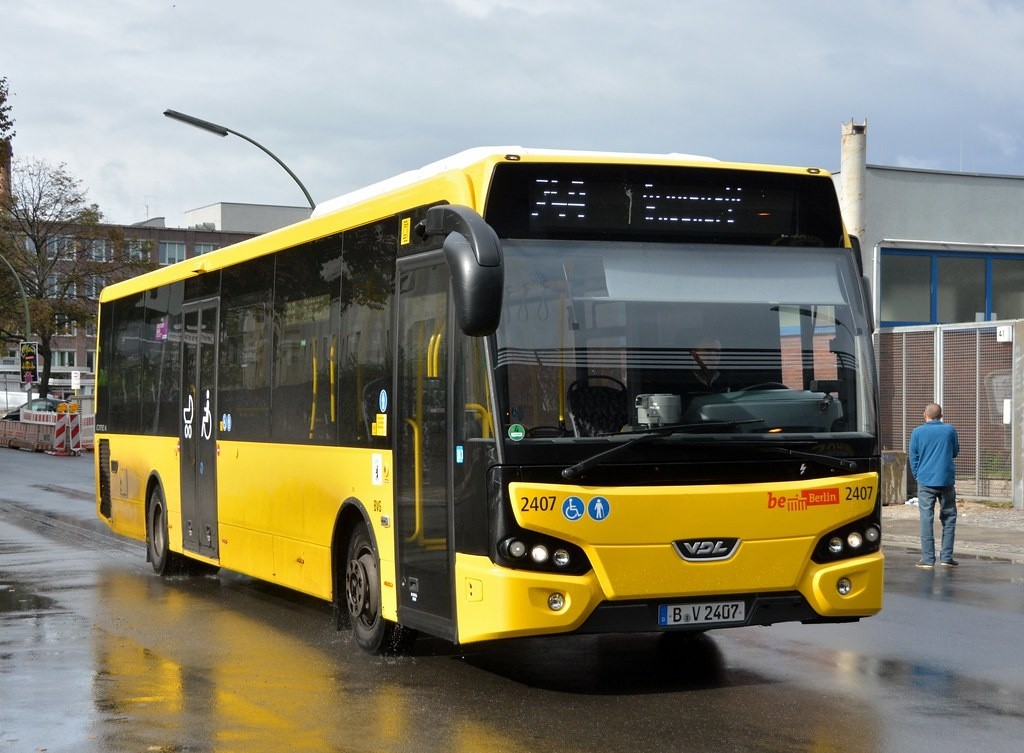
[566, 376, 627, 438]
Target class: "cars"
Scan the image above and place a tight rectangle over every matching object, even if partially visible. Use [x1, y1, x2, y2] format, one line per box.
[3, 396, 70, 421]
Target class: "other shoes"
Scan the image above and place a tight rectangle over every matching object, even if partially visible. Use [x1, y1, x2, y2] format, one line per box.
[915, 559, 935, 568]
[940, 558, 960, 568]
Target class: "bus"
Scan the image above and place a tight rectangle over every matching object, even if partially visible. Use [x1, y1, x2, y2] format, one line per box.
[84, 146, 887, 658]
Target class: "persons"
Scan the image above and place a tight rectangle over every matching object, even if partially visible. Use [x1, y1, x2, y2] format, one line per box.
[909, 403, 959, 567]
[673, 335, 750, 412]
